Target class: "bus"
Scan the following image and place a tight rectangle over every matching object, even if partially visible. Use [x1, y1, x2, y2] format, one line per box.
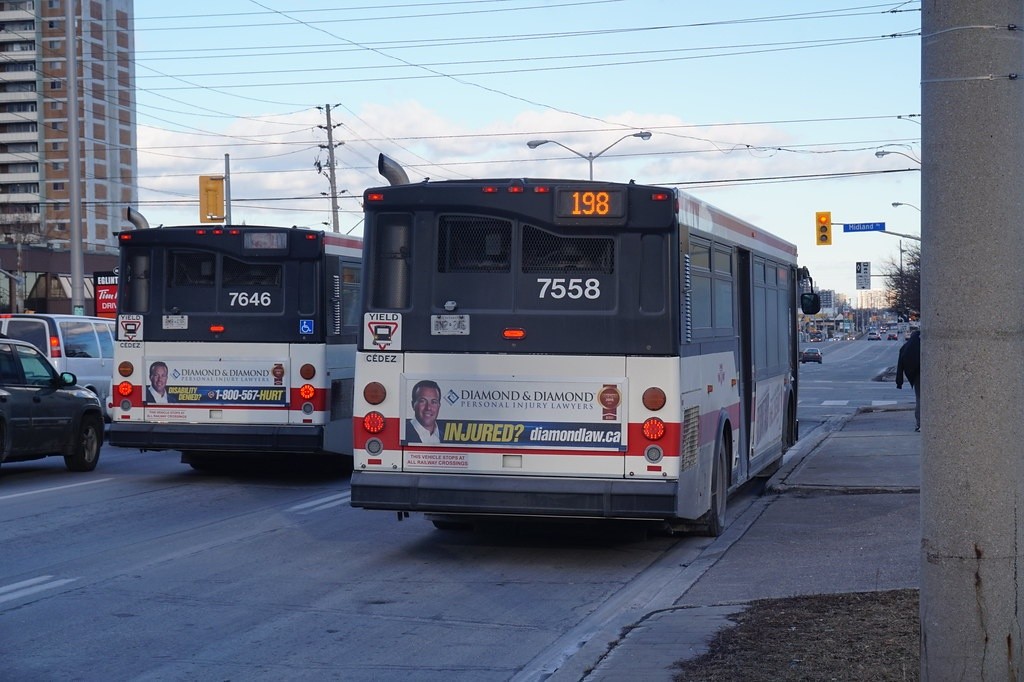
[810, 330, 825, 342]
[346, 153, 821, 537]
[107, 205, 365, 474]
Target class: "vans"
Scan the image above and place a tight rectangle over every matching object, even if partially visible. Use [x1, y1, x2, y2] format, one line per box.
[0, 314, 117, 425]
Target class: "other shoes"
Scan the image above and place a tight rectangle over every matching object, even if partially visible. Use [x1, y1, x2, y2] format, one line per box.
[915, 424, 921, 432]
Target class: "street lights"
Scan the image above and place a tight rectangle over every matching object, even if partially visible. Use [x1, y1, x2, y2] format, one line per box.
[527, 131, 653, 182]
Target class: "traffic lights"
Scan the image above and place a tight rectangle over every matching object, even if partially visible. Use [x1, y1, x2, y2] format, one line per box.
[816, 212, 832, 245]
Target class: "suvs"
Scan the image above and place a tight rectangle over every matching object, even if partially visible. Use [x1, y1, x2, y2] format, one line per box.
[0, 333, 105, 472]
[868, 332, 878, 340]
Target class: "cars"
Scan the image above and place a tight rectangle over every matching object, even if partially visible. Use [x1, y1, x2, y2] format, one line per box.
[887, 333, 898, 340]
[881, 322, 914, 339]
[802, 348, 823, 364]
[833, 336, 840, 340]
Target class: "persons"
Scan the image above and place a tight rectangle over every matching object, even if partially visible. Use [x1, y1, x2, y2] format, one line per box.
[146, 361, 176, 403]
[896, 330, 920, 432]
[405, 381, 444, 443]
